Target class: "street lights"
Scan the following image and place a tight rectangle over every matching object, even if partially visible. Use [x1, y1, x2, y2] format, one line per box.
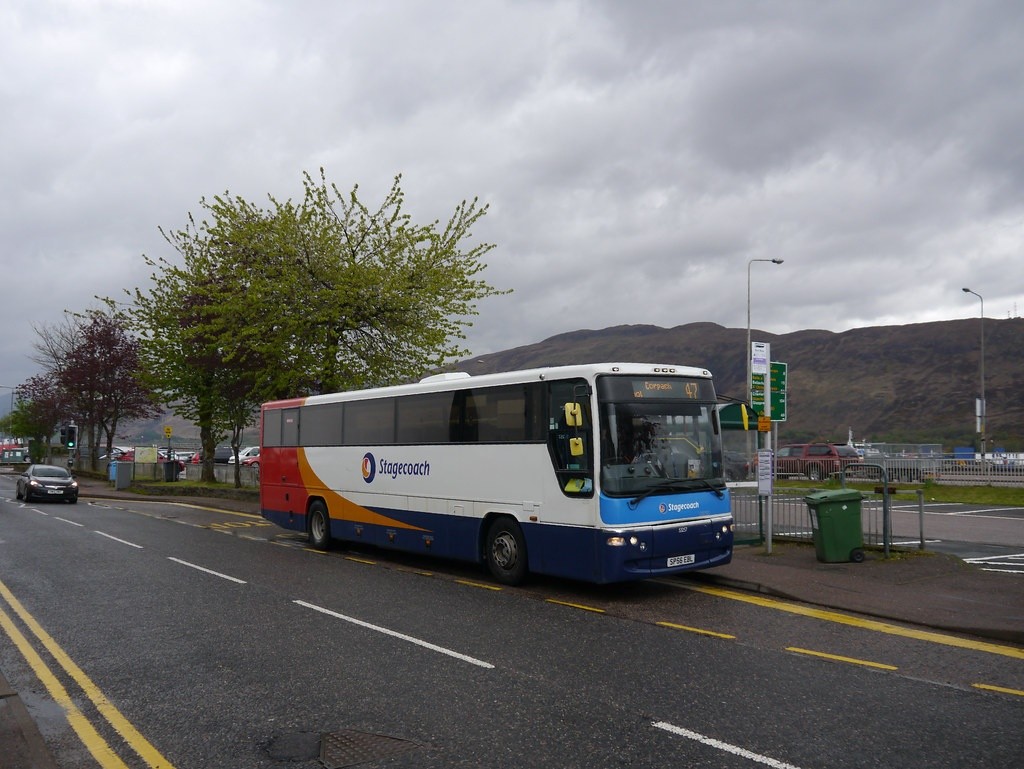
[0, 385, 16, 465]
[748, 257, 785, 481]
[961, 286, 987, 475]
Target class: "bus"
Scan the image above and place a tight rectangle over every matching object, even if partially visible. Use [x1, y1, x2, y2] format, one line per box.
[259, 360, 752, 589]
[0, 435, 50, 464]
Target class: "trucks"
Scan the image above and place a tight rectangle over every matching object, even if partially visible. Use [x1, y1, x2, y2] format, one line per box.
[853, 442, 941, 482]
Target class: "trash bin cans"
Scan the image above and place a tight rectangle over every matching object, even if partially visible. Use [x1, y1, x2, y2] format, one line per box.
[109, 461, 117, 480]
[801, 489, 869, 564]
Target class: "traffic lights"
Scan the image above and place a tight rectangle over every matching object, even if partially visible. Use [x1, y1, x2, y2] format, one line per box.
[61, 426, 78, 449]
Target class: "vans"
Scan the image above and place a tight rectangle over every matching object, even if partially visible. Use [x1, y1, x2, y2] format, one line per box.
[190, 446, 241, 464]
[775, 443, 860, 480]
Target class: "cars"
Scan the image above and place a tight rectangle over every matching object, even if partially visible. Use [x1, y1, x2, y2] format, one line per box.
[16, 464, 79, 505]
[228, 445, 260, 467]
[724, 449, 749, 480]
[80, 445, 193, 472]
